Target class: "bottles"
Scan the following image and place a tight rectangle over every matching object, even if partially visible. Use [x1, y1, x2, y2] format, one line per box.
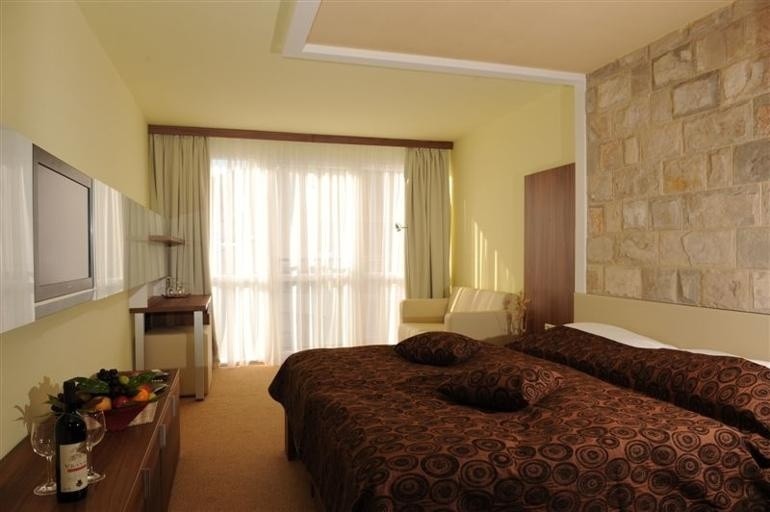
[52, 381, 88, 506]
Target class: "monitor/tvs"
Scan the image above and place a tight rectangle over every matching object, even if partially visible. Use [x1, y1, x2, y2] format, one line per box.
[32, 143, 95, 319]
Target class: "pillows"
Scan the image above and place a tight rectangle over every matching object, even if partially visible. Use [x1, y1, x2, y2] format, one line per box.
[431, 360, 565, 413]
[508, 319, 770, 435]
[392, 331, 484, 366]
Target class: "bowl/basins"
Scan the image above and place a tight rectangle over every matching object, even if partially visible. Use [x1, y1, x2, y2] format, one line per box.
[51, 384, 169, 433]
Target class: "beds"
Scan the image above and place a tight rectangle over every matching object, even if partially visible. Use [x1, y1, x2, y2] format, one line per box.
[267, 291, 770, 512]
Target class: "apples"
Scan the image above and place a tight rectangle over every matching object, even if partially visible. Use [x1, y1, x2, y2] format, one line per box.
[112, 396, 129, 408]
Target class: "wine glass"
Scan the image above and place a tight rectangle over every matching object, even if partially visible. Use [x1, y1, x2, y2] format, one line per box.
[29, 419, 58, 496]
[81, 410, 107, 483]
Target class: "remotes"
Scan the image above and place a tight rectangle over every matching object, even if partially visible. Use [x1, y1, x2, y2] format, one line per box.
[127, 372, 168, 380]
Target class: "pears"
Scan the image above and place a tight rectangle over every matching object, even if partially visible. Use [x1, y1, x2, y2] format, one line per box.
[90, 396, 112, 411]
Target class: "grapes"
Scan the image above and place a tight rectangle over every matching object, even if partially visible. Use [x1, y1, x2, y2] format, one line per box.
[96, 368, 127, 398]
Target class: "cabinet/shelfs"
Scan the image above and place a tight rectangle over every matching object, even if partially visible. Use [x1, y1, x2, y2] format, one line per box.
[0, 367, 180, 512]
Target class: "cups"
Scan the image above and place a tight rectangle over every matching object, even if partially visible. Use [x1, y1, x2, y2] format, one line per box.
[164, 275, 185, 296]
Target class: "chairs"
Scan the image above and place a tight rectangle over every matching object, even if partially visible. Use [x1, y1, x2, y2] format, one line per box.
[398, 285, 516, 342]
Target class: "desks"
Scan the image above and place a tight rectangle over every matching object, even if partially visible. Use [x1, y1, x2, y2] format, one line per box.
[129, 295, 216, 400]
